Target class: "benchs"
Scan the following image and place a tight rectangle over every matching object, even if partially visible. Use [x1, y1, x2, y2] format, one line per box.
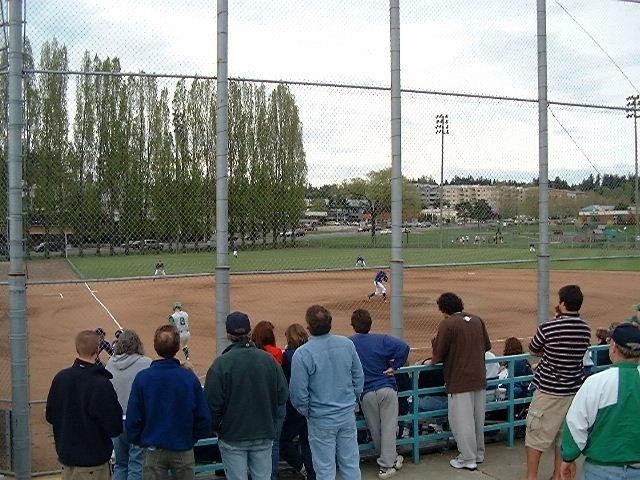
[192, 346, 616, 474]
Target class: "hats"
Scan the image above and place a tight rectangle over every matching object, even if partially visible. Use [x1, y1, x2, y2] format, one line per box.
[225, 310, 251, 336]
[607, 323, 640, 351]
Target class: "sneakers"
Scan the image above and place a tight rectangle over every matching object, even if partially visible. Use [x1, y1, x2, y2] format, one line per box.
[377, 467, 397, 479]
[449, 456, 478, 472]
[395, 453, 404, 469]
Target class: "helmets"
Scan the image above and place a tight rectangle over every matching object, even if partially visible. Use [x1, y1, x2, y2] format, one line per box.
[173, 301, 183, 310]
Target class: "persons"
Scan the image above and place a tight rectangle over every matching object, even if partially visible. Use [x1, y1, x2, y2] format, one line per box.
[356, 255, 365, 268]
[503, 336, 533, 400]
[455, 233, 504, 245]
[366, 270, 388, 303]
[409, 338, 448, 436]
[104, 328, 155, 480]
[126, 324, 212, 480]
[554, 305, 594, 379]
[281, 324, 316, 480]
[169, 301, 190, 361]
[347, 309, 410, 479]
[529, 244, 536, 253]
[289, 304, 365, 480]
[394, 361, 412, 417]
[431, 292, 491, 471]
[179, 361, 218, 463]
[251, 320, 286, 480]
[233, 247, 238, 258]
[46, 331, 122, 480]
[521, 284, 592, 480]
[95, 328, 113, 370]
[559, 323, 640, 480]
[498, 362, 509, 401]
[153, 260, 167, 281]
[590, 327, 613, 365]
[112, 330, 122, 350]
[608, 322, 621, 330]
[204, 311, 289, 480]
[485, 351, 501, 403]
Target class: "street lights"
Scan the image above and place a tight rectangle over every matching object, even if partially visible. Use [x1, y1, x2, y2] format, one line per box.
[626, 94, 640, 243]
[435, 113, 449, 232]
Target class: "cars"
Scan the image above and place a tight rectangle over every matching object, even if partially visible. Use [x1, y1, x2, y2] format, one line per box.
[496, 215, 539, 228]
[229, 235, 238, 242]
[121, 239, 165, 249]
[302, 218, 360, 231]
[35, 242, 65, 252]
[358, 220, 439, 235]
[243, 233, 260, 242]
[280, 229, 306, 238]
